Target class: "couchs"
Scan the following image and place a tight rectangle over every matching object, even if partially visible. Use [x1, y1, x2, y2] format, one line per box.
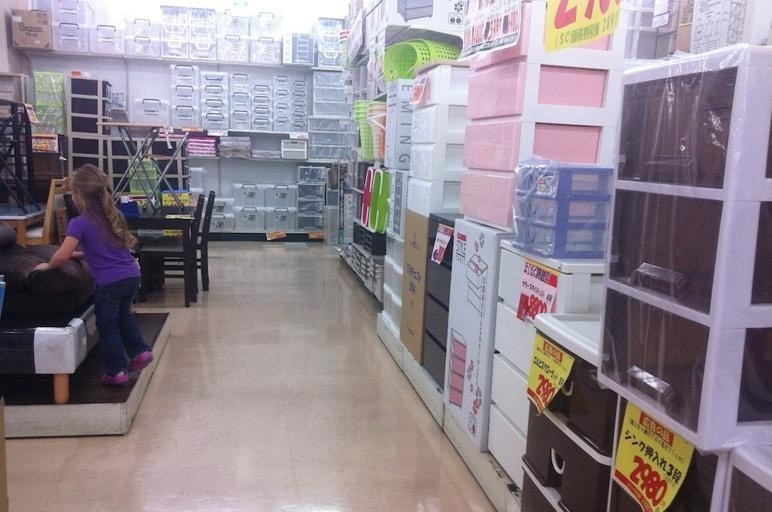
[0, 221, 100, 405]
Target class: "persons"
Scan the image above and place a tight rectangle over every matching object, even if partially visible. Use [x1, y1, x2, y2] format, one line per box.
[32, 163, 153, 386]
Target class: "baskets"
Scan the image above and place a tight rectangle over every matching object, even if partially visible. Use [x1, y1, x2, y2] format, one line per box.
[353, 100, 378, 161]
[367, 102, 386, 160]
[382, 38, 461, 82]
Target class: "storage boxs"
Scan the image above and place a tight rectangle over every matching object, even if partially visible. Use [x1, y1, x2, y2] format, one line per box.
[185, 132, 354, 234]
[132, 65, 357, 136]
[39, 73, 111, 178]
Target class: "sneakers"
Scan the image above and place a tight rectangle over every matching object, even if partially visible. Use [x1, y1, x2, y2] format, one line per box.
[98, 369, 129, 386]
[130, 349, 156, 372]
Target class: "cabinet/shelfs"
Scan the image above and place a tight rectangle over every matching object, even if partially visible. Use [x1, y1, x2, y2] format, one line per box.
[1, 98, 43, 214]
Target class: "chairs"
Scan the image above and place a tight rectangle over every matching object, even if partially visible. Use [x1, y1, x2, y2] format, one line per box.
[161, 190, 216, 291]
[25, 176, 68, 245]
[139, 194, 206, 307]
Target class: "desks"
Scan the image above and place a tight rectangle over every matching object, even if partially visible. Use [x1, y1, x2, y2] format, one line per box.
[0, 205, 46, 247]
[96, 122, 203, 209]
[118, 200, 200, 307]
